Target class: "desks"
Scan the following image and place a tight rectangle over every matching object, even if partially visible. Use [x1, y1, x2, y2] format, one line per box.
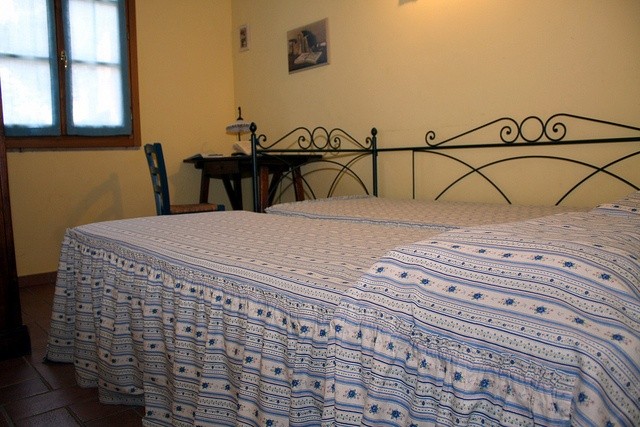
[183, 155, 323, 212]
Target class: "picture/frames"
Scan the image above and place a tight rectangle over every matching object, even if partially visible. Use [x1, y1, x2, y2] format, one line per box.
[285, 17, 330, 75]
[237, 24, 251, 53]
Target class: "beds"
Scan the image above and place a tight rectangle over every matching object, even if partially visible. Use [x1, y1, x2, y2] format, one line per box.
[46, 113, 639, 426]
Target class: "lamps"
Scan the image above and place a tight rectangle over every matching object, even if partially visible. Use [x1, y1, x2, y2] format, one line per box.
[226, 107, 250, 155]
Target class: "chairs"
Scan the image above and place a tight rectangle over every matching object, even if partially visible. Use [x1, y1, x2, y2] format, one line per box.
[144, 142, 224, 214]
